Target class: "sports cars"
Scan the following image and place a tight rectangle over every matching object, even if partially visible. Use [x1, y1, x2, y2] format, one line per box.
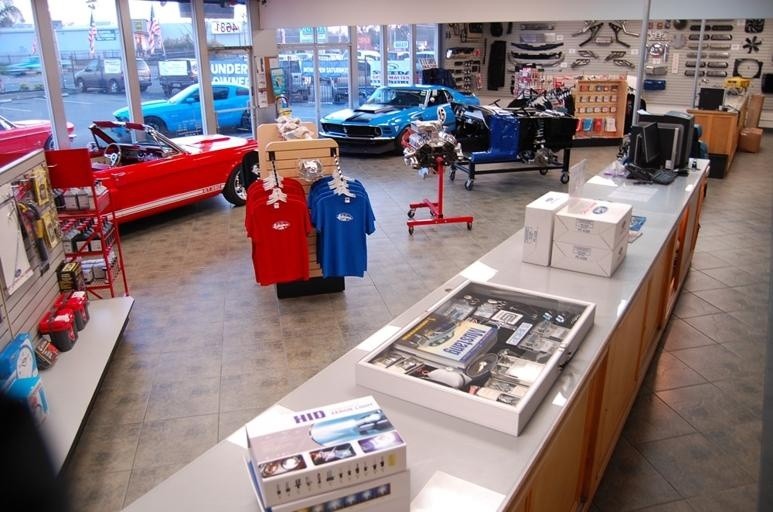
[73, 118, 257, 229]
[316, 80, 482, 160]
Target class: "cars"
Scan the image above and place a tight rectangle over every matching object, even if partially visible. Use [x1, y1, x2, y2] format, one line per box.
[111, 81, 252, 142]
[157, 36, 436, 104]
[5, 53, 71, 76]
[0, 112, 76, 170]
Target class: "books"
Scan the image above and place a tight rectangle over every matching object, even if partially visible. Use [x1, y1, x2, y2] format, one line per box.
[390, 313, 497, 373]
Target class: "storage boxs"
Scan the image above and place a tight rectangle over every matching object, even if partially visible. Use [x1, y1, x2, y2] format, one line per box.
[2, 331, 48, 429]
[37, 287, 91, 352]
[58, 261, 89, 304]
[242, 395, 411, 512]
[522, 190, 633, 278]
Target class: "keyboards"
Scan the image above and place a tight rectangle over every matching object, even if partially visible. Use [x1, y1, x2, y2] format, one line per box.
[652, 169, 678, 185]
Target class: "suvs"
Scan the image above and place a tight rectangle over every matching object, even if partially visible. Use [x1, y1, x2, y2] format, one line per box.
[72, 56, 152, 93]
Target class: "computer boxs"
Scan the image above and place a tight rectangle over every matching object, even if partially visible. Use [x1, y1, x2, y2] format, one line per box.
[635, 109, 695, 170]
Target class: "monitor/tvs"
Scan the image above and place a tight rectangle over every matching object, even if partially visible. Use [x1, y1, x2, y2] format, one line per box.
[626, 122, 661, 181]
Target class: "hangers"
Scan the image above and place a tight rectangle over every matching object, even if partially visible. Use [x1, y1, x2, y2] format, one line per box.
[263, 159, 288, 206]
[328, 154, 356, 199]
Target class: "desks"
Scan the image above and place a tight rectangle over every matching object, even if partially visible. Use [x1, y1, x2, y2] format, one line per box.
[743, 94, 765, 128]
[687, 91, 749, 175]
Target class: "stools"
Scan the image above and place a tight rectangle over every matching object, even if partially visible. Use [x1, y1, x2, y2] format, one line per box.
[739, 127, 763, 153]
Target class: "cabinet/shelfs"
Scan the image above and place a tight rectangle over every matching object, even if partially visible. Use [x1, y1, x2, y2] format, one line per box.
[44, 146, 129, 299]
[257, 121, 346, 298]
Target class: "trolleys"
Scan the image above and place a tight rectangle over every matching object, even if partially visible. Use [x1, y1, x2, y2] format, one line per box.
[449, 106, 579, 191]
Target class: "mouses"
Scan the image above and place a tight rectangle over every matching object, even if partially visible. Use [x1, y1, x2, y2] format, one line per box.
[678, 168, 689, 176]
